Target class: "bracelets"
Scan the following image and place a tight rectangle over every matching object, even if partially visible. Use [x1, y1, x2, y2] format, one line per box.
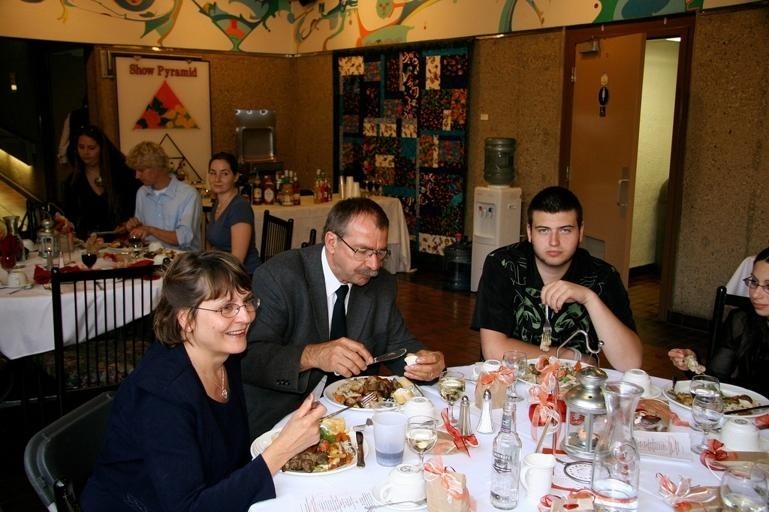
[569, 343, 582, 361]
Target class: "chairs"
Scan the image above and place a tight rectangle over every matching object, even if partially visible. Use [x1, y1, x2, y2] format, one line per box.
[24, 389, 118, 512]
[48, 263, 164, 427]
[191, 204, 315, 265]
[701, 284, 750, 363]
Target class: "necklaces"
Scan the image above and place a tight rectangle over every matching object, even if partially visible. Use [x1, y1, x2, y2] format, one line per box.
[216, 197, 228, 212]
[88, 169, 105, 186]
[189, 344, 228, 399]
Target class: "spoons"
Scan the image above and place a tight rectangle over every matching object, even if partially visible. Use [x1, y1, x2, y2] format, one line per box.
[9, 283, 37, 296]
[356, 498, 429, 512]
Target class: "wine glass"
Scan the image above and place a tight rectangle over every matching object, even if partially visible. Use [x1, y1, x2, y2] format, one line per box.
[718, 464, 769, 512]
[687, 373, 724, 457]
[81, 250, 98, 272]
[502, 350, 527, 403]
[406, 411, 440, 472]
[438, 371, 465, 427]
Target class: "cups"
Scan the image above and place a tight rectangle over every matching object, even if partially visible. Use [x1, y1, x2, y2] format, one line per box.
[379, 464, 428, 503]
[7, 269, 29, 287]
[518, 450, 559, 505]
[621, 367, 653, 400]
[719, 416, 762, 454]
[337, 173, 363, 202]
[471, 358, 502, 381]
[370, 411, 408, 469]
[406, 396, 434, 418]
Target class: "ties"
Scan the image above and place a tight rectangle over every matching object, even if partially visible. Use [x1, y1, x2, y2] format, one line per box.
[322, 284, 352, 394]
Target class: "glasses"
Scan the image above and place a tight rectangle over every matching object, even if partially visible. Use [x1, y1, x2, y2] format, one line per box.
[741, 274, 769, 294]
[327, 228, 390, 263]
[177, 294, 266, 320]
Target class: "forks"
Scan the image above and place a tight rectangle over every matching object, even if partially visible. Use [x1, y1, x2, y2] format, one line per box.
[540, 302, 555, 346]
[308, 389, 375, 424]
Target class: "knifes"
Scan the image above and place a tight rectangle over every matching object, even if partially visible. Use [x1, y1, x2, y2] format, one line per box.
[722, 403, 769, 416]
[353, 426, 367, 470]
[331, 346, 407, 377]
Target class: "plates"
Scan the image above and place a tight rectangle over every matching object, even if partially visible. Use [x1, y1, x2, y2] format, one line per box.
[369, 480, 431, 511]
[515, 358, 590, 389]
[323, 375, 418, 414]
[662, 379, 768, 421]
[0, 281, 33, 291]
[106, 239, 157, 253]
[247, 432, 369, 476]
[614, 380, 662, 401]
[469, 375, 511, 386]
[702, 438, 769, 488]
[138, 249, 194, 266]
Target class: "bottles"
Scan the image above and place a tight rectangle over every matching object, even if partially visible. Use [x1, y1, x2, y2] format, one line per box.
[458, 388, 522, 510]
[591, 379, 644, 506]
[46, 250, 65, 270]
[0, 214, 29, 270]
[364, 179, 387, 199]
[35, 213, 76, 258]
[236, 164, 335, 207]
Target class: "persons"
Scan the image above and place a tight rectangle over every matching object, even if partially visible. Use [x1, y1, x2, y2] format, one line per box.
[123, 142, 204, 255]
[231, 196, 449, 432]
[52, 127, 134, 246]
[83, 250, 326, 511]
[668, 245, 769, 399]
[472, 184, 645, 371]
[205, 151, 262, 271]
